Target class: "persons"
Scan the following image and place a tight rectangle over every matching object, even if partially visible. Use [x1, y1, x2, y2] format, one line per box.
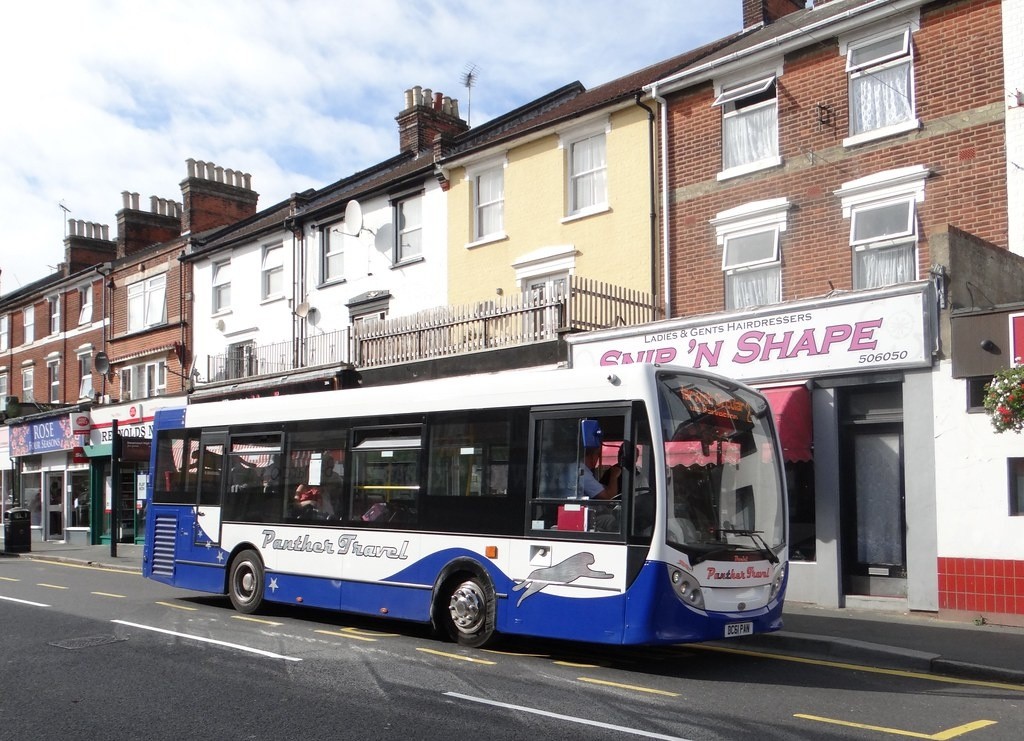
[292, 455, 343, 522]
[599, 442, 651, 537]
[559, 441, 621, 532]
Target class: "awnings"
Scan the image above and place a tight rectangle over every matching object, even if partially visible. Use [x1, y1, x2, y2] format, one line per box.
[592, 388, 812, 469]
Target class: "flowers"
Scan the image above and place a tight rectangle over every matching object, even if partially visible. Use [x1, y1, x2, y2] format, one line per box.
[981, 356, 1024, 436]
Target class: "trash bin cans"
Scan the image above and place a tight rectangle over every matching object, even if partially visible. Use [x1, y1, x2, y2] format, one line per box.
[3, 506, 32, 554]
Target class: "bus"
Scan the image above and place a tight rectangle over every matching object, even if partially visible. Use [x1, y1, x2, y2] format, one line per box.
[142, 361, 791, 649]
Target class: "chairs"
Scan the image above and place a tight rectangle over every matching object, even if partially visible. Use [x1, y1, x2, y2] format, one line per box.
[364, 493, 385, 514]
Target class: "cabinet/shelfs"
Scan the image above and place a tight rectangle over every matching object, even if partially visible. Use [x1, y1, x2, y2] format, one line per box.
[116, 469, 134, 544]
